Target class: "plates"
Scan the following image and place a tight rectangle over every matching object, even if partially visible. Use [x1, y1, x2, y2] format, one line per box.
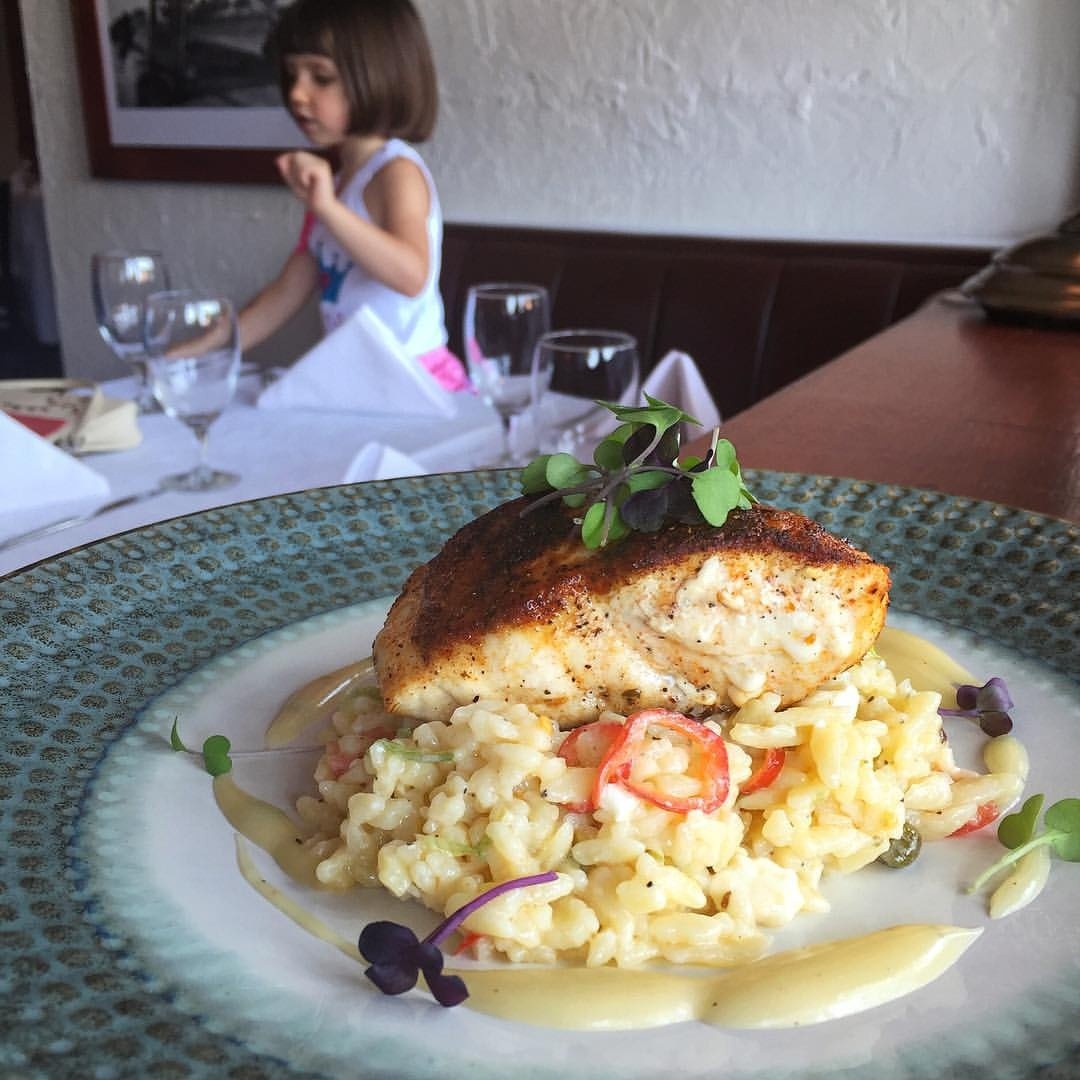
[0, 475, 1080, 1080]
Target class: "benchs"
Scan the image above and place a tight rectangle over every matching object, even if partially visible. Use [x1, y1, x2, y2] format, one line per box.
[440, 223, 1000, 430]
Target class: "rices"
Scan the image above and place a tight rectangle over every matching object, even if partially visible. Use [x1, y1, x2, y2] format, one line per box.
[295, 649, 961, 964]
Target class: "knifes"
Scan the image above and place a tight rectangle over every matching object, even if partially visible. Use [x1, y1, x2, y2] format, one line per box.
[0, 492, 164, 551]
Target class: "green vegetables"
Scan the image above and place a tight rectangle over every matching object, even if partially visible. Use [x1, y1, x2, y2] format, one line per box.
[171, 677, 1080, 1006]
[516, 390, 760, 547]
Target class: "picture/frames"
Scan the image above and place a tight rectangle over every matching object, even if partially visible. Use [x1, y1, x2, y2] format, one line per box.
[67, 0, 340, 182]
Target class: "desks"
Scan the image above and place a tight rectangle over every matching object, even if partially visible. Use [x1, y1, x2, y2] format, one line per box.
[681, 290, 1080, 532]
[0, 382, 600, 579]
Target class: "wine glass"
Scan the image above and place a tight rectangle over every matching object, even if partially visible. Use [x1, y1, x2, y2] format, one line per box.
[90, 245, 169, 414]
[463, 284, 550, 469]
[145, 289, 242, 491]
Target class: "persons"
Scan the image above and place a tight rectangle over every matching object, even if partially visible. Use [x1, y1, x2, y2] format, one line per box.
[165, 1, 468, 396]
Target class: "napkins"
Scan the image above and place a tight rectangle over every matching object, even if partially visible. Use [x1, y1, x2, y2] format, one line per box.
[252, 307, 456, 424]
[639, 346, 721, 441]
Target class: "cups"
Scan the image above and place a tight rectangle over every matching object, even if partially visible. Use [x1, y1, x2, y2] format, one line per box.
[531, 330, 639, 468]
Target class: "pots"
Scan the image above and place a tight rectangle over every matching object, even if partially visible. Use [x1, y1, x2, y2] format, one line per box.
[958, 212, 1080, 325]
